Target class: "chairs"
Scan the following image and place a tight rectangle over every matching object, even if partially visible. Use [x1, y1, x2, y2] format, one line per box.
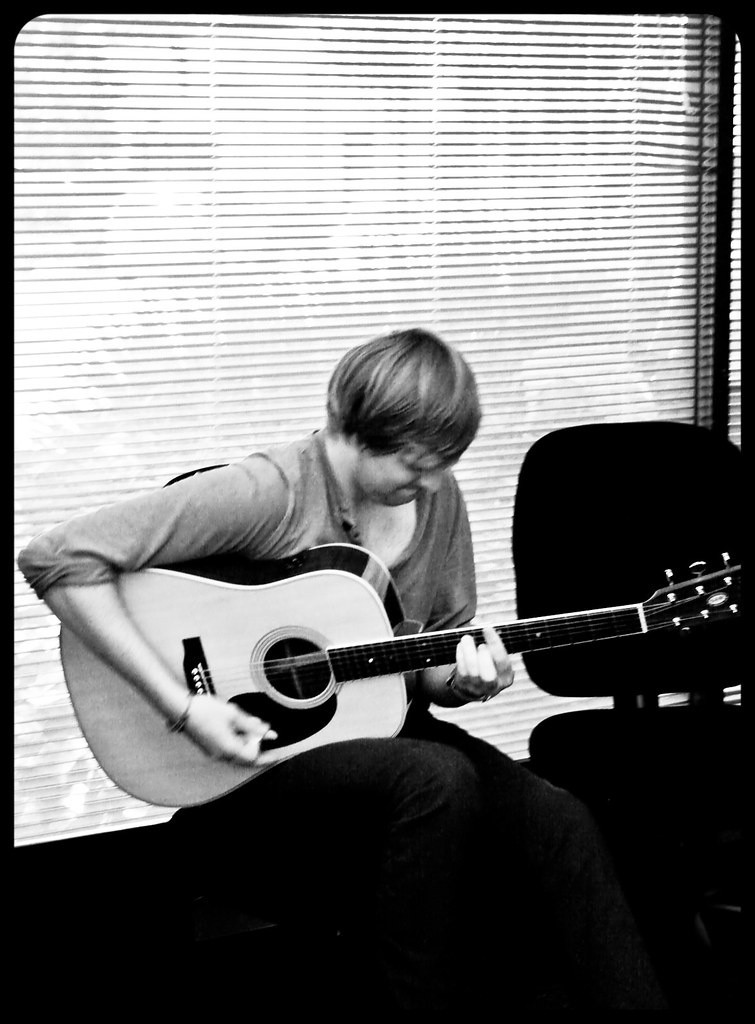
[512, 420, 755, 1024]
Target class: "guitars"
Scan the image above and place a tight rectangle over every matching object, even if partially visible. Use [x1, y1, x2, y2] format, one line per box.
[57, 543, 742, 809]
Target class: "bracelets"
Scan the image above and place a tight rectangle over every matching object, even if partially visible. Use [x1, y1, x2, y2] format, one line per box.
[170, 694, 194, 733]
[445, 669, 489, 702]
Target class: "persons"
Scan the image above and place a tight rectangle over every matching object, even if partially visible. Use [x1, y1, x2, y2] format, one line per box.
[18, 327, 667, 1012]
